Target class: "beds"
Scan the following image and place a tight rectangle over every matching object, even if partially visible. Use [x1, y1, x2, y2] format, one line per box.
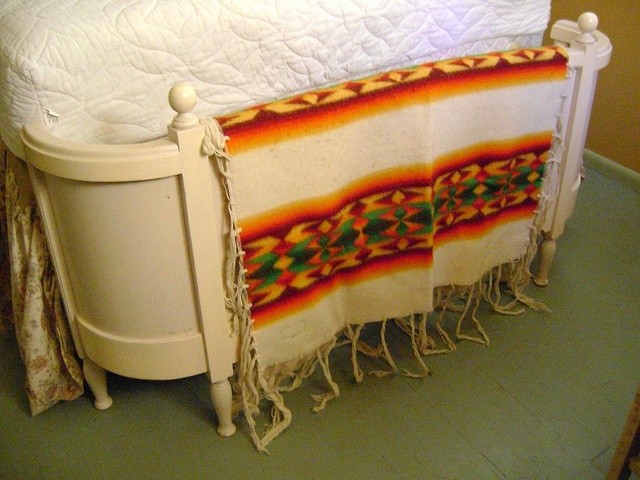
[1, 2, 613, 439]
